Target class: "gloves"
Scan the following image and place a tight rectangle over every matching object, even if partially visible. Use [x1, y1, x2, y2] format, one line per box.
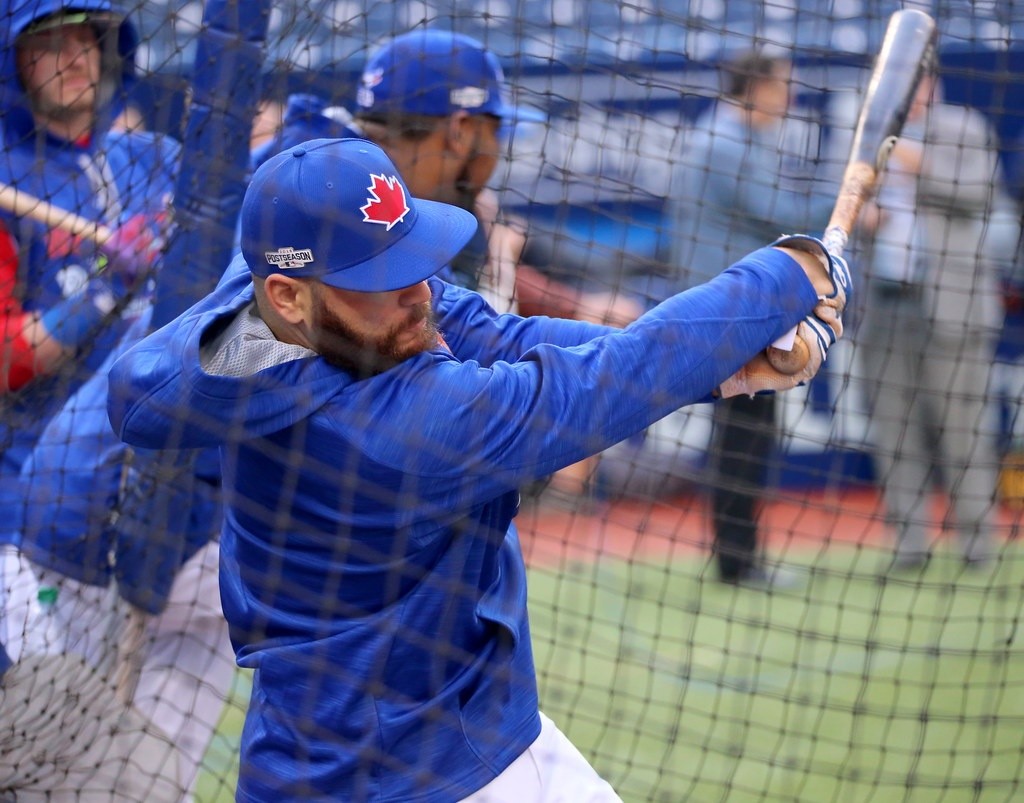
[715, 234, 853, 399]
[42, 210, 182, 346]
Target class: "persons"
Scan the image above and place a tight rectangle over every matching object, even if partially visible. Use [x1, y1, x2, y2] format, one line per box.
[215, 134, 855, 802]
[668, 41, 890, 596]
[862, 41, 1024, 580]
[3, 0, 648, 803]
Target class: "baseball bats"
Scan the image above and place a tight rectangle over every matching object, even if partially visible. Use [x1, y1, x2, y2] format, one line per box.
[0, 181, 118, 249]
[764, 6, 939, 375]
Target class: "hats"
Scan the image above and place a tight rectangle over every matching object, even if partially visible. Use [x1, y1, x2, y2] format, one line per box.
[16, 8, 124, 35]
[356, 30, 547, 124]
[240, 138, 478, 293]
